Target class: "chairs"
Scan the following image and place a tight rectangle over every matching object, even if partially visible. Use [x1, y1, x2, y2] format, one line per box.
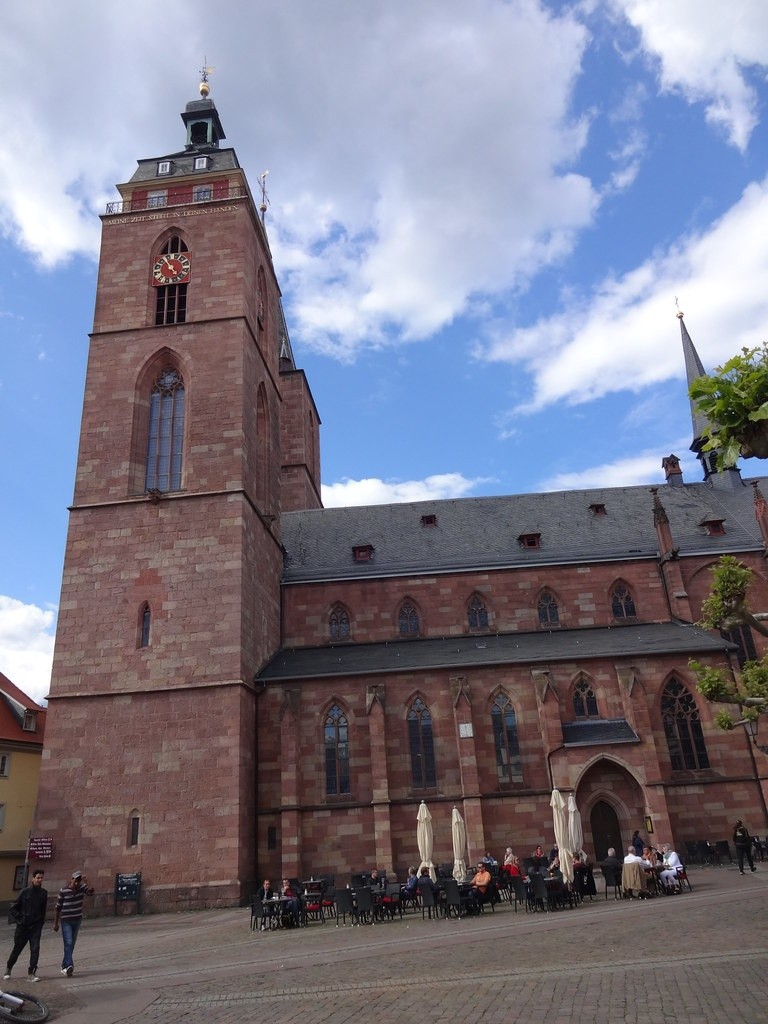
[687, 834, 768, 866]
[250, 857, 694, 933]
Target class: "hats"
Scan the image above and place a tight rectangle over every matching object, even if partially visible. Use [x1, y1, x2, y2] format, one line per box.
[72, 872, 83, 878]
[506, 847, 513, 853]
[736, 820, 742, 824]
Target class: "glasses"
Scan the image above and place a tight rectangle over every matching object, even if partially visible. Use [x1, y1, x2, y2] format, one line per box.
[478, 867, 482, 868]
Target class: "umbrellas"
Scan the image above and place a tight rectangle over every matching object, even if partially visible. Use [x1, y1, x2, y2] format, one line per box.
[415, 799, 438, 887]
[549, 785, 588, 892]
[450, 805, 467, 886]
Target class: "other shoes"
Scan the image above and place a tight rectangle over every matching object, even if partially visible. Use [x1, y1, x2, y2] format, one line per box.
[752, 867, 756, 873]
[3, 971, 11, 980]
[27, 975, 40, 982]
[61, 968, 67, 975]
[67, 967, 74, 977]
[740, 872, 744, 874]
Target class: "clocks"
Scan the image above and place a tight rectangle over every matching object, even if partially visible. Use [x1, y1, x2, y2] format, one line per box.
[459, 723, 473, 738]
[152, 251, 191, 286]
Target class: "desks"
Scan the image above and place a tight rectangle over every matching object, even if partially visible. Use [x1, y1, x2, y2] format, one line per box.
[642, 863, 669, 873]
[301, 878, 328, 891]
[261, 895, 296, 928]
[437, 881, 474, 891]
[301, 892, 321, 898]
[351, 890, 387, 896]
[522, 873, 559, 885]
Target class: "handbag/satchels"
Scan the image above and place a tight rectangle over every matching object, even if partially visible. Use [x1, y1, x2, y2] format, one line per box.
[281, 915, 295, 929]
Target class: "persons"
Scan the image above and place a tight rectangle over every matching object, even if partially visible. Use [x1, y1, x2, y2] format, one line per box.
[3, 869, 49, 983]
[52, 871, 95, 977]
[368, 869, 381, 886]
[482, 852, 497, 867]
[530, 846, 547, 860]
[503, 848, 517, 866]
[255, 878, 303, 932]
[526, 865, 550, 904]
[604, 843, 684, 898]
[420, 866, 447, 915]
[733, 820, 756, 875]
[391, 866, 419, 915]
[632, 829, 645, 857]
[460, 861, 492, 916]
[509, 856, 528, 881]
[547, 842, 597, 896]
